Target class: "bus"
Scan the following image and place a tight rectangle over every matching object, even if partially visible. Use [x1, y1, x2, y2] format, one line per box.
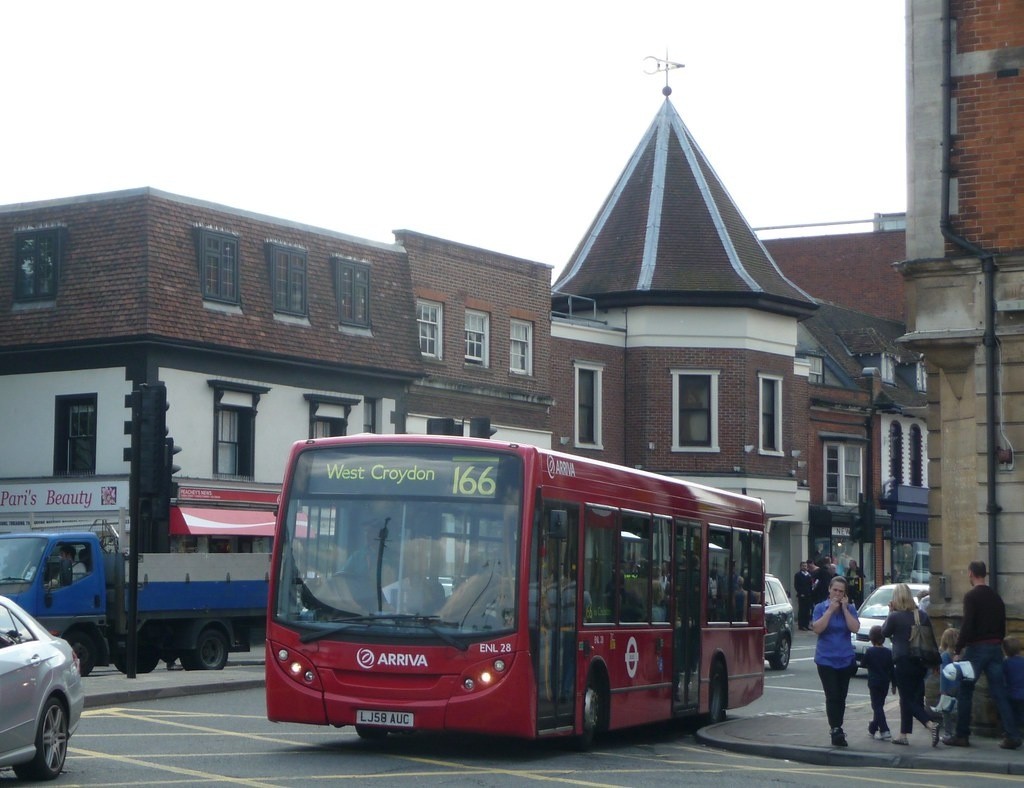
[264, 431, 769, 753]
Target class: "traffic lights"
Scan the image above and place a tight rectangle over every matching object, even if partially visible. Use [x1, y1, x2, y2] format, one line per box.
[851, 513, 862, 542]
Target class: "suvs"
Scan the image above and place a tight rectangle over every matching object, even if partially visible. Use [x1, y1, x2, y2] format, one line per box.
[761, 572, 794, 670]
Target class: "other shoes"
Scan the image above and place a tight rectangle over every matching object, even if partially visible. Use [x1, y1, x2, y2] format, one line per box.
[830, 727, 848, 746]
[1000, 736, 1024, 749]
[892, 738, 909, 745]
[931, 722, 940, 747]
[799, 626, 811, 631]
[942, 736, 969, 746]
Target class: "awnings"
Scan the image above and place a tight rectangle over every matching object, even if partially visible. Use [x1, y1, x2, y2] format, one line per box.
[170, 505, 316, 539]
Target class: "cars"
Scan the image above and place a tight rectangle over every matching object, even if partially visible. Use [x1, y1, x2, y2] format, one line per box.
[0, 595, 84, 782]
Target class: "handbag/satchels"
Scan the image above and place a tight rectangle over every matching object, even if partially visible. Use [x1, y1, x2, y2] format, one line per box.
[908, 609, 933, 662]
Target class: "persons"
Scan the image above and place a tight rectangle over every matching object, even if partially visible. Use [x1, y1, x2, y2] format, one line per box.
[590, 568, 665, 623]
[812, 576, 860, 747]
[929, 560, 1024, 749]
[465, 561, 509, 626]
[547, 567, 592, 702]
[861, 625, 897, 740]
[792, 554, 867, 632]
[49, 546, 87, 586]
[882, 583, 943, 748]
[671, 541, 765, 701]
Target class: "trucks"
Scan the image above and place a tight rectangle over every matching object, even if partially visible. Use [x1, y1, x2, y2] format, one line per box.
[0, 530, 273, 677]
[911, 541, 931, 584]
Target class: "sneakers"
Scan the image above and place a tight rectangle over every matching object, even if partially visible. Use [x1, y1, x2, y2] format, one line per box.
[868, 721, 875, 735]
[874, 730, 891, 740]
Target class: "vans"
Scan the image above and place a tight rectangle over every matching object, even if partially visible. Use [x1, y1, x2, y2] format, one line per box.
[849, 581, 929, 675]
